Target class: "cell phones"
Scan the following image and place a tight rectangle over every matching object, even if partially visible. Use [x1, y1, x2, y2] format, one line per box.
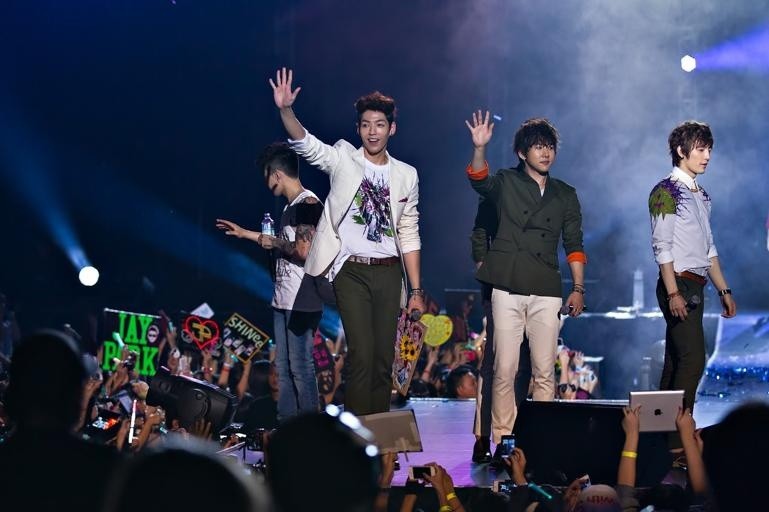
[91, 413, 122, 435]
[493, 480, 519, 492]
[528, 483, 552, 503]
[408, 466, 435, 481]
[501, 435, 517, 460]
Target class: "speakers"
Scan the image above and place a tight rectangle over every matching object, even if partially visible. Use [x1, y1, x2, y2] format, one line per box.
[504, 399, 672, 485]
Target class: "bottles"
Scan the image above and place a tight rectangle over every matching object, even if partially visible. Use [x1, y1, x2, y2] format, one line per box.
[262, 212, 275, 236]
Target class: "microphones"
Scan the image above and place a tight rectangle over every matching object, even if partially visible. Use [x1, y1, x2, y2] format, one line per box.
[668, 295, 701, 327]
[408, 290, 422, 322]
[560, 306, 588, 315]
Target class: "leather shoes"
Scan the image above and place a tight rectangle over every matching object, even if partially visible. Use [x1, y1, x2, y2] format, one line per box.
[473, 438, 507, 468]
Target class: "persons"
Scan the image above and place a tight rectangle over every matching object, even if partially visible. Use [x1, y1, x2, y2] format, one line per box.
[267, 66, 427, 417]
[463, 109, 586, 460]
[649, 120, 738, 422]
[1, 281, 768, 511]
[471, 190, 532, 464]
[215, 141, 329, 421]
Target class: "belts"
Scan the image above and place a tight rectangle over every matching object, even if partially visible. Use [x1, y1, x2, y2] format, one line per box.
[346, 255, 401, 266]
[674, 271, 707, 286]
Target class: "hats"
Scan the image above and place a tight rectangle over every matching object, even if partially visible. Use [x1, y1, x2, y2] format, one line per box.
[579, 484, 620, 510]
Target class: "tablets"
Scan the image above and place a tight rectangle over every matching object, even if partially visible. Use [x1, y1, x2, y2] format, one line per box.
[628, 389, 685, 432]
